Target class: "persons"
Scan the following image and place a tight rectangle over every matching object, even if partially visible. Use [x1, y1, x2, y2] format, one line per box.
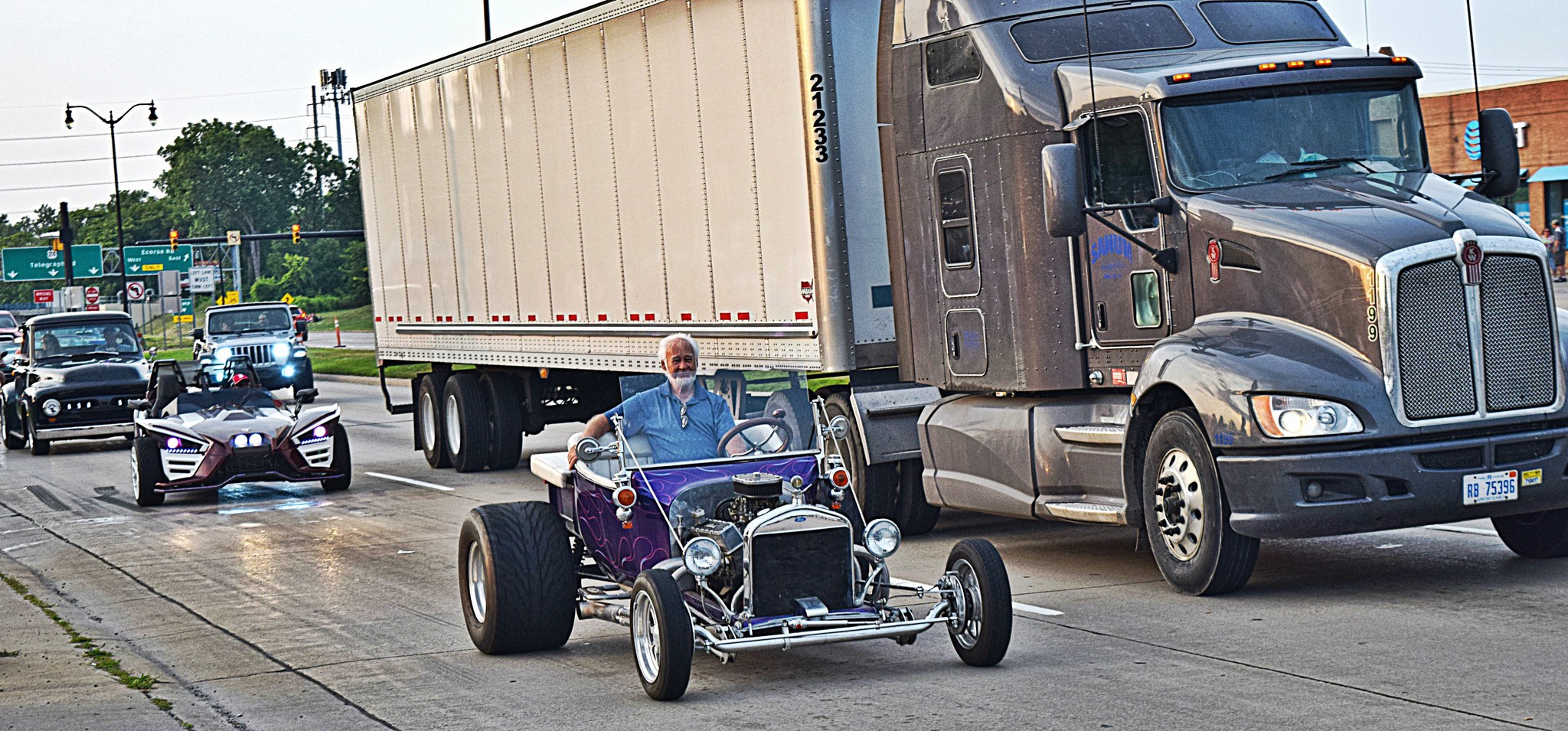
[220, 317, 236, 331]
[1254, 109, 1328, 179]
[226, 374, 252, 407]
[306, 313, 320, 323]
[36, 334, 63, 359]
[1542, 229, 1558, 280]
[95, 326, 136, 352]
[1551, 220, 1567, 282]
[258, 313, 281, 329]
[567, 333, 748, 471]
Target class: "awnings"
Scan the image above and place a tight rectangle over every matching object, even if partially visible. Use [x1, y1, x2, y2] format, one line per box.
[1526, 166, 1568, 182]
[1461, 168, 1527, 187]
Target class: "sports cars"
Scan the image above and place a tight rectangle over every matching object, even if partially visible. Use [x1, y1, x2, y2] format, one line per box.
[127, 354, 353, 507]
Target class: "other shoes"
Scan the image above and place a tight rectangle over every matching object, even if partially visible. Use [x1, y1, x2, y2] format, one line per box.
[1553, 276, 1567, 283]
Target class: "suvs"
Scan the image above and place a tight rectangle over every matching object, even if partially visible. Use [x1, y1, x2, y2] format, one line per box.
[0, 311, 158, 456]
[190, 301, 316, 404]
[288, 304, 308, 341]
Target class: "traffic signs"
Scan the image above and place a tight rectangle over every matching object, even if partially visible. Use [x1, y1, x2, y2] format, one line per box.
[2, 244, 103, 282]
[122, 244, 194, 277]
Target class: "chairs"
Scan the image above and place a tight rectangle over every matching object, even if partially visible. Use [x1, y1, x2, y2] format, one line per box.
[219, 356, 262, 408]
[146, 359, 189, 418]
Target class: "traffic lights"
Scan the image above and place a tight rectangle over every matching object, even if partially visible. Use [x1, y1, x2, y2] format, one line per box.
[169, 230, 178, 250]
[291, 224, 301, 244]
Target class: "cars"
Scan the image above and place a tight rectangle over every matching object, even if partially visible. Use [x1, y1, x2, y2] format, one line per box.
[0, 310, 107, 389]
[459, 368, 1013, 703]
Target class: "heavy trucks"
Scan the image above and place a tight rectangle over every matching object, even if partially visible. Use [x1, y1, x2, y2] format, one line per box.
[350, 0, 1568, 598]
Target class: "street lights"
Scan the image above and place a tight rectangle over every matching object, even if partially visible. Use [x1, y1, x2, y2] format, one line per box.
[319, 67, 348, 232]
[63, 97, 161, 312]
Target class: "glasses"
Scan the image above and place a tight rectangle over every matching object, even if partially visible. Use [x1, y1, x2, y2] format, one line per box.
[680, 403, 688, 428]
[1544, 231, 1550, 232]
[41, 339, 53, 343]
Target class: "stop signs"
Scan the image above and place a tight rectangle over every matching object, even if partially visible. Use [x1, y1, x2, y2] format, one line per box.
[85, 286, 100, 305]
